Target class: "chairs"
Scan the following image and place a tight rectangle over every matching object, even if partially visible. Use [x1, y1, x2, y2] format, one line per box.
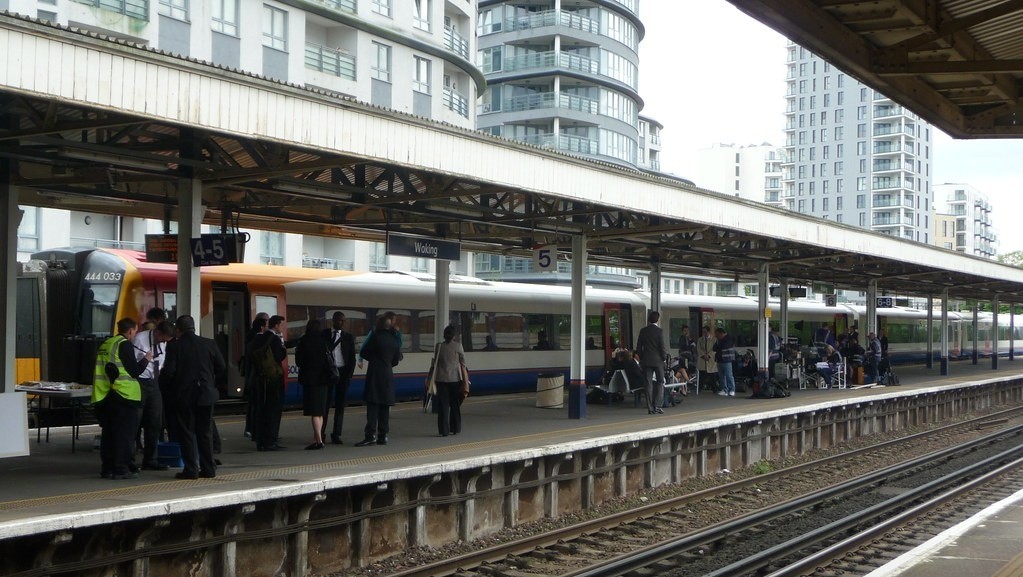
[591, 364, 700, 407]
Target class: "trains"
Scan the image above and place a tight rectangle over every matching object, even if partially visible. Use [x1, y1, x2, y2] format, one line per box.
[16, 245, 1023, 411]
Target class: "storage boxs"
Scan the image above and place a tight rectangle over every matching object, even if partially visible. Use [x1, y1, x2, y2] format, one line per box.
[774, 363, 789, 375]
[157, 440, 186, 468]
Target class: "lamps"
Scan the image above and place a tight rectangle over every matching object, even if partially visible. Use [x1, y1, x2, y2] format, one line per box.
[56, 145, 168, 172]
[536, 220, 583, 234]
[271, 180, 352, 199]
[424, 201, 484, 218]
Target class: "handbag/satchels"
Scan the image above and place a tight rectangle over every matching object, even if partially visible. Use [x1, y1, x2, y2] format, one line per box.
[425, 343, 441, 395]
[319, 337, 337, 370]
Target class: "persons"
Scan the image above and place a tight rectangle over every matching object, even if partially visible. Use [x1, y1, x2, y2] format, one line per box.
[636, 311, 667, 415]
[482, 335, 499, 349]
[424, 325, 467, 435]
[533, 330, 552, 350]
[585, 323, 737, 396]
[240, 311, 403, 453]
[767, 322, 888, 390]
[90, 307, 228, 480]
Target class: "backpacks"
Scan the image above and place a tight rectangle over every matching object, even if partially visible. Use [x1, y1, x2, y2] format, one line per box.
[249, 335, 284, 381]
[758, 376, 791, 398]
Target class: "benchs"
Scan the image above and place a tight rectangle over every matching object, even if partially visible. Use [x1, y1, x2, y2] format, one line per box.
[796, 351, 846, 389]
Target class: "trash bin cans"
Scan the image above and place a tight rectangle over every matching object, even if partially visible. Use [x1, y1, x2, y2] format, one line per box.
[536, 371, 564, 409]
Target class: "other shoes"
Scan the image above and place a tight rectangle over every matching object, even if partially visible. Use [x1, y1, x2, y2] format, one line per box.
[331, 435, 344, 445]
[717, 389, 728, 396]
[257, 444, 289, 451]
[729, 391, 735, 396]
[101, 469, 111, 479]
[354, 437, 376, 447]
[377, 437, 387, 445]
[112, 470, 139, 479]
[141, 462, 170, 471]
[304, 442, 324, 451]
[199, 471, 216, 478]
[175, 472, 198, 479]
[212, 449, 221, 454]
[244, 431, 252, 437]
[648, 406, 664, 414]
[132, 465, 141, 471]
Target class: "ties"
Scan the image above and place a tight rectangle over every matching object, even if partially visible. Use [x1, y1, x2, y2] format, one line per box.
[153, 344, 159, 380]
[333, 331, 338, 341]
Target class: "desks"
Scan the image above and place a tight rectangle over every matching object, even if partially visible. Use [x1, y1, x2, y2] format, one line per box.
[14, 381, 94, 452]
